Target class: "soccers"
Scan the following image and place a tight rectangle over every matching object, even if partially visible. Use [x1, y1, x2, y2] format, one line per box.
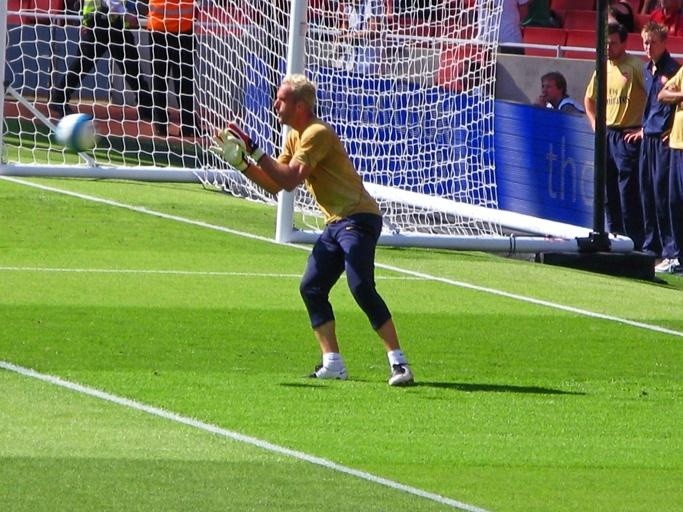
[56, 113, 98, 152]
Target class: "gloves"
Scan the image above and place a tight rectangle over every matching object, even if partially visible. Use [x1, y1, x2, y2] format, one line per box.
[209, 122, 266, 173]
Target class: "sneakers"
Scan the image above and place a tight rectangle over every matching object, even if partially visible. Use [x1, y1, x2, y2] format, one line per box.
[388, 363, 414, 387]
[308, 365, 349, 380]
[136, 107, 206, 137]
[654, 258, 680, 273]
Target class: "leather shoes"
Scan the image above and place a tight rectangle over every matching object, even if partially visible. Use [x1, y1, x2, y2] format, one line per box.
[50, 101, 74, 117]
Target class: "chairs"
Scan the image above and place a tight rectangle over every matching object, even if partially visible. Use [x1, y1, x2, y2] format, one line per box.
[387, 0, 683, 61]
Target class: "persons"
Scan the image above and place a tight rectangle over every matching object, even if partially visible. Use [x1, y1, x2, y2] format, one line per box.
[328, 1, 564, 89]
[536, 74, 586, 116]
[209, 74, 414, 386]
[586, 1, 683, 277]
[50, 0, 197, 135]
